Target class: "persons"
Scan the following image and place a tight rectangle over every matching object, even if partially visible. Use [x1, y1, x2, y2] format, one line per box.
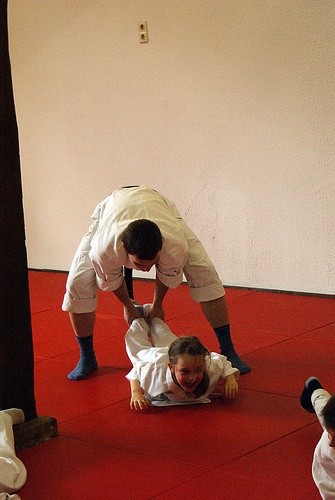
[124, 303, 240, 411]
[301, 376, 335, 499]
[0, 408, 27, 500]
[61, 184, 251, 381]
